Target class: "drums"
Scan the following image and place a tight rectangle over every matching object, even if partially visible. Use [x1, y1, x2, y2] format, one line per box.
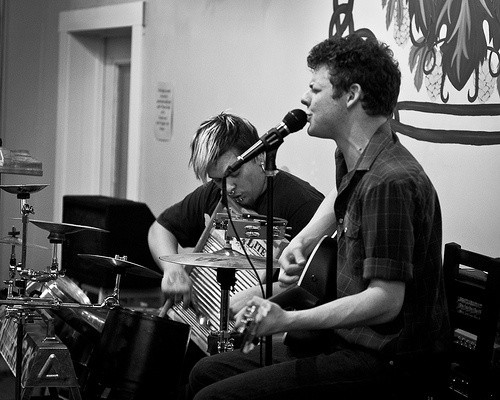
[25, 270, 92, 320]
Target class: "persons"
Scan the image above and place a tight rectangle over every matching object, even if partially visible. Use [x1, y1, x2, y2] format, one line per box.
[187, 33, 443, 400]
[147, 111, 325, 358]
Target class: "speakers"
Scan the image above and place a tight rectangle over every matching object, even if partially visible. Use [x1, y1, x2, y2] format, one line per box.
[61, 194, 163, 292]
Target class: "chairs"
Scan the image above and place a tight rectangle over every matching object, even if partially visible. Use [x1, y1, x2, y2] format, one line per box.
[443, 243, 500, 400]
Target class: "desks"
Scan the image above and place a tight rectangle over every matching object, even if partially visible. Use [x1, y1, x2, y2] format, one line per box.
[75, 281, 161, 305]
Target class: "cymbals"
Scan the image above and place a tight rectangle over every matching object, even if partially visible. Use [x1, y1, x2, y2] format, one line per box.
[159, 250, 282, 268]
[216, 211, 293, 240]
[11, 218, 110, 236]
[73, 253, 161, 277]
[0, 183, 50, 194]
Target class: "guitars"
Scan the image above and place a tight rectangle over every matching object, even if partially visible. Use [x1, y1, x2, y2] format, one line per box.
[207, 230, 338, 355]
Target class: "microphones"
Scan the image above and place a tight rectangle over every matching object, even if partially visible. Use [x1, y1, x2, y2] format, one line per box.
[227, 109, 307, 173]
